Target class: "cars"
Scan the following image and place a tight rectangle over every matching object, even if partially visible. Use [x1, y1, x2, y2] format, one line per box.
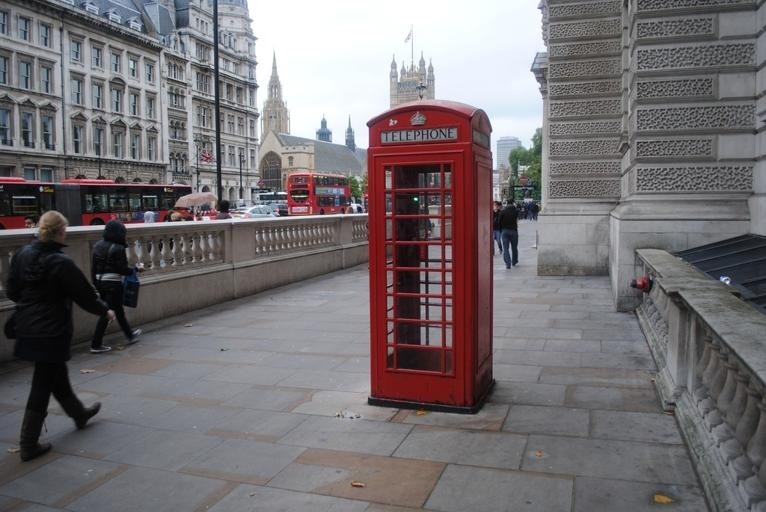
[231, 204, 276, 219]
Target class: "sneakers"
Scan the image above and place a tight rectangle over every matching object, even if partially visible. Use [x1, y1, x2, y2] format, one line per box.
[89, 345, 112, 353]
[127, 328, 142, 344]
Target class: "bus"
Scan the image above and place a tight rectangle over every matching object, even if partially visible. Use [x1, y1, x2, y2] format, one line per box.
[257, 192, 288, 213]
[0, 176, 194, 253]
[365, 185, 368, 210]
[287, 173, 353, 216]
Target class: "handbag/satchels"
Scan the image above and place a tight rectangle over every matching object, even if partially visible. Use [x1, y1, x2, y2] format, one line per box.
[3, 309, 20, 340]
[123, 271, 140, 308]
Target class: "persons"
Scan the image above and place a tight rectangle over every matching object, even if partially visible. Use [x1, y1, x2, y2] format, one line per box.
[20, 215, 40, 227]
[3, 210, 118, 463]
[88, 219, 146, 353]
[492, 199, 542, 255]
[499, 199, 521, 270]
[417, 202, 435, 259]
[141, 199, 233, 250]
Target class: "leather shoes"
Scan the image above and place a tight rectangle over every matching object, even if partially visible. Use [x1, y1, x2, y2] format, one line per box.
[76, 401, 101, 429]
[19, 442, 52, 462]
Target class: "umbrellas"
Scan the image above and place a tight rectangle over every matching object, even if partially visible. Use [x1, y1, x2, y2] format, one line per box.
[173, 192, 217, 211]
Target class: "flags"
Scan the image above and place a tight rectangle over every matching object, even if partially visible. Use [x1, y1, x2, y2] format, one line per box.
[200, 149, 215, 165]
[404, 30, 413, 44]
